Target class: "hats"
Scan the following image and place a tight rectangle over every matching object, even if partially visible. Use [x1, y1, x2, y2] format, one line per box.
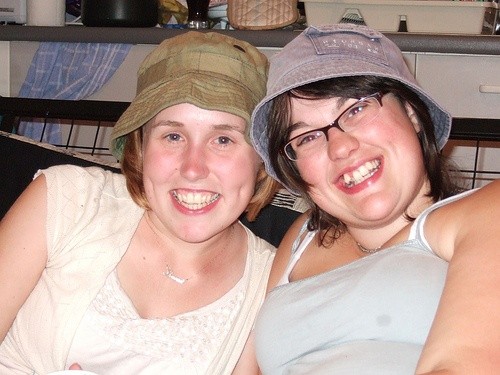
[110, 31, 270, 162]
[250, 23, 451, 196]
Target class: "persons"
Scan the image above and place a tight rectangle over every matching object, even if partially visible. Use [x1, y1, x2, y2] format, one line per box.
[249, 21, 500, 375]
[1, 30, 278, 375]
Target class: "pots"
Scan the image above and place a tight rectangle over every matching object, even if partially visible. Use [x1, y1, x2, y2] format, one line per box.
[81, 0, 157, 26]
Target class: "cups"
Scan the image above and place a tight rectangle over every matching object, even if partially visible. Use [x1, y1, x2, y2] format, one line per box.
[186, 0, 210, 28]
[26, 0, 66, 26]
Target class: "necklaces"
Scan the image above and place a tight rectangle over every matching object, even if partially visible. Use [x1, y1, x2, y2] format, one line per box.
[355, 241, 380, 252]
[144, 209, 234, 284]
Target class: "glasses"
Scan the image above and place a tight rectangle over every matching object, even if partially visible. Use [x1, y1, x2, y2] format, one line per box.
[282, 91, 391, 162]
